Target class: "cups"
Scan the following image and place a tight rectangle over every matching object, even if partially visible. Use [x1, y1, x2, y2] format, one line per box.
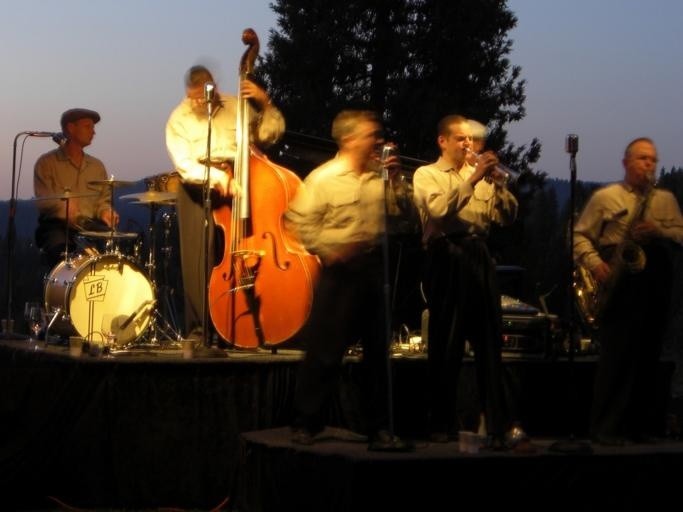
[1, 319, 14, 333]
[458, 432, 480, 454]
[69, 336, 83, 357]
[180, 339, 195, 359]
[579, 338, 591, 351]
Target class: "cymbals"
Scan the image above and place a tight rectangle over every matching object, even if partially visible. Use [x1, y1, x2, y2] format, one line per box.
[25, 188, 102, 202]
[85, 178, 136, 191]
[77, 226, 140, 241]
[118, 187, 178, 206]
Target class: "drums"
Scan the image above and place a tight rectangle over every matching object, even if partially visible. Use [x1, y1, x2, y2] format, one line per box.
[44, 248, 158, 346]
[142, 171, 181, 200]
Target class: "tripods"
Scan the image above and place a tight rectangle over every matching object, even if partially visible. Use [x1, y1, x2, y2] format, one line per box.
[128, 232, 186, 346]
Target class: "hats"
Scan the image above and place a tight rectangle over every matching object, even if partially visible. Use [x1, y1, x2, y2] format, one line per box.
[61, 110, 99, 128]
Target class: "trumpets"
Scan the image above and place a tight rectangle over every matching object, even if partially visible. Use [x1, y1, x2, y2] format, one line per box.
[464, 144, 525, 189]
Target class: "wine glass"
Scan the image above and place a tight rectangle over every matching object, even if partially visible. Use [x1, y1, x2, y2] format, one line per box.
[22, 303, 47, 353]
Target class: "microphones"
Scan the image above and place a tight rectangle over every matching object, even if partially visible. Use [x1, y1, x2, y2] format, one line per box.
[121, 301, 148, 329]
[203, 83, 216, 116]
[564, 133, 579, 171]
[29, 131, 68, 146]
[382, 142, 397, 183]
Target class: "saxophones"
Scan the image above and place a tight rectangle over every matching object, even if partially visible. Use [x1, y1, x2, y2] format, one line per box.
[571, 169, 656, 333]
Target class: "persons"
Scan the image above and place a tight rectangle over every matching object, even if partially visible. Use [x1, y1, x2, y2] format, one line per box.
[565, 139, 683, 445]
[164, 64, 288, 336]
[28, 107, 124, 347]
[411, 114, 529, 448]
[278, 108, 414, 441]
[463, 119, 490, 158]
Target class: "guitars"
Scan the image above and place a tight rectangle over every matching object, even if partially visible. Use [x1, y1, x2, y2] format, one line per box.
[208, 27, 322, 350]
